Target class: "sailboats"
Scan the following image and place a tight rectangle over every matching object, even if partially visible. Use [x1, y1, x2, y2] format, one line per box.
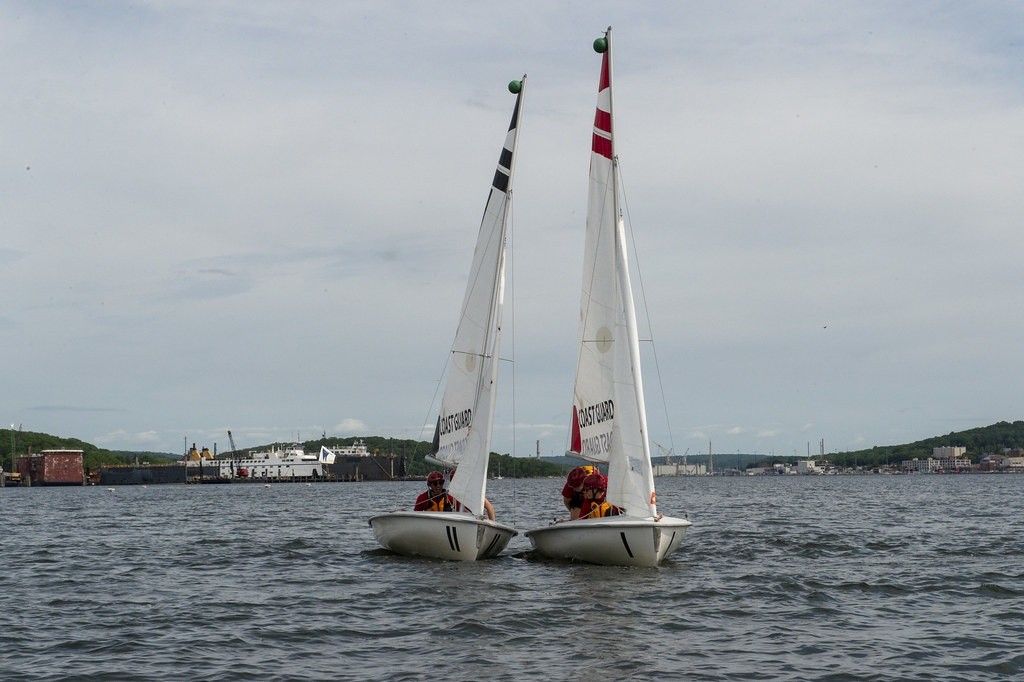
[364, 72, 538, 558]
[529, 22, 694, 572]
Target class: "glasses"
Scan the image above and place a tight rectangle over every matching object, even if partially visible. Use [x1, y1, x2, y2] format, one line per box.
[432, 481, 445, 486]
[582, 487, 596, 492]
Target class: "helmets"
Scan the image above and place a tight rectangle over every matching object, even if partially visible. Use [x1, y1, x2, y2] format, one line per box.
[567, 468, 586, 488]
[450, 468, 456, 482]
[427, 471, 445, 486]
[583, 473, 607, 491]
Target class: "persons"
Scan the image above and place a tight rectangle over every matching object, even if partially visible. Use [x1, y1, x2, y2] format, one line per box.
[562, 466, 624, 519]
[414, 471, 495, 521]
